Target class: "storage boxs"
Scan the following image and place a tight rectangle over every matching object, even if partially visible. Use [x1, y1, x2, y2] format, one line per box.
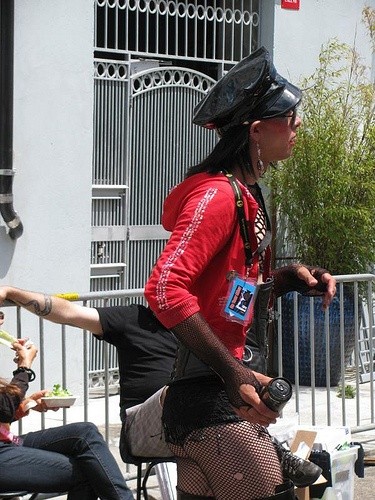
[329, 445, 360, 500]
[294, 430, 327, 500]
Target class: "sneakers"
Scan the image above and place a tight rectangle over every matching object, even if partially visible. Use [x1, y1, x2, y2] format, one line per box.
[273, 440, 323, 489]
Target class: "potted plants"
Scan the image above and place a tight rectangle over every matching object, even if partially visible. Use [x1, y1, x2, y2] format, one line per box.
[261, 4, 375, 387]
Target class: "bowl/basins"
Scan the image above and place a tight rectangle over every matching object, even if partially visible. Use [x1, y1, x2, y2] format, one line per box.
[41, 396, 76, 408]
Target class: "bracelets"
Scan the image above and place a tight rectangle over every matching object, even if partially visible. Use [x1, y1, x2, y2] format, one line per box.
[13, 366, 36, 382]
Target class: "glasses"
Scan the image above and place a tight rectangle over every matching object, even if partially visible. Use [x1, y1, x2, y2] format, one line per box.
[281, 108, 297, 127]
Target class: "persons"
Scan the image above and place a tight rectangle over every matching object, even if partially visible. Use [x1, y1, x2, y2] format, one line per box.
[143, 47, 337, 500]
[0, 311, 134, 500]
[0, 285, 323, 500]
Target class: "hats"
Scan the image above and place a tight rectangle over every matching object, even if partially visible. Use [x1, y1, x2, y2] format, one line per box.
[192, 46, 302, 137]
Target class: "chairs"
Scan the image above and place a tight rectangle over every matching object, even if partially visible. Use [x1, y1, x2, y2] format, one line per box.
[118, 409, 176, 500]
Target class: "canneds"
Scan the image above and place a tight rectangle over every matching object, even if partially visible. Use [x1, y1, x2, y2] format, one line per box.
[260, 377, 292, 413]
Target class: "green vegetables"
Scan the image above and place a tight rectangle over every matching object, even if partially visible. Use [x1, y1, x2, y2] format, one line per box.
[52, 384, 70, 395]
